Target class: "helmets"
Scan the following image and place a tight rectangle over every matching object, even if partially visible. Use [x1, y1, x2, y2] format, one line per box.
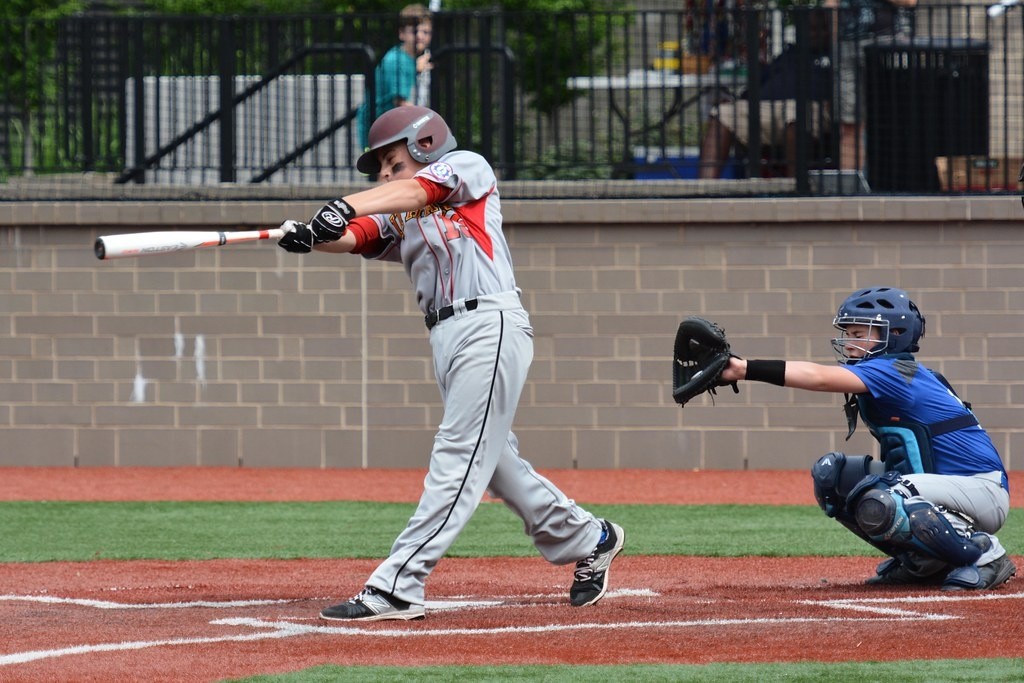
[835, 284, 926, 358]
[358, 105, 458, 174]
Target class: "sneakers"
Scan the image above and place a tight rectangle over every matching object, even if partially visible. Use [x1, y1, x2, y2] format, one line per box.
[942, 537, 1016, 591]
[569, 518, 626, 609]
[319, 586, 426, 622]
[867, 554, 946, 582]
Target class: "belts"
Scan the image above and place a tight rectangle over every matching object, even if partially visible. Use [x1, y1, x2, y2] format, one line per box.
[424, 295, 480, 329]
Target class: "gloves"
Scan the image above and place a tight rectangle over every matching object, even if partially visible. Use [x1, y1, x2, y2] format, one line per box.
[276, 219, 314, 253]
[312, 198, 356, 242]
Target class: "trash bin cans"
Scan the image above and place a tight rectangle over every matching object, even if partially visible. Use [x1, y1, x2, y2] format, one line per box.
[864, 37, 991, 192]
[628, 143, 738, 180]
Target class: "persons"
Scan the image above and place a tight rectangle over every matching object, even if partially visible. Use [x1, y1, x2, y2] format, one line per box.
[671, 286, 1016, 592]
[693, 0, 920, 180]
[357, 2, 436, 168]
[273, 105, 626, 622]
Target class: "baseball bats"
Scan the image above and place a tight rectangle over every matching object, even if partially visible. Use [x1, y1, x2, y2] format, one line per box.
[94, 222, 347, 260]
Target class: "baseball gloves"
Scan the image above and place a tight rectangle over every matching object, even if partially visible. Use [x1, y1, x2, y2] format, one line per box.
[670, 316, 743, 406]
